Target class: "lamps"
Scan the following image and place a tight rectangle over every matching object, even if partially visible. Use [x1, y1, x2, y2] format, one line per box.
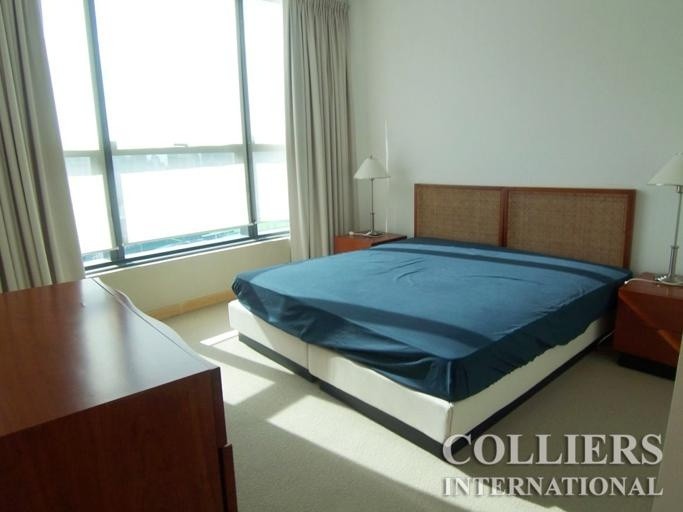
[353, 154, 391, 236]
[646, 153, 683, 286]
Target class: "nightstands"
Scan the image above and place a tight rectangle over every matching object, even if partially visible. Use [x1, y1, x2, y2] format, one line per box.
[333, 230, 407, 254]
[612, 272, 683, 380]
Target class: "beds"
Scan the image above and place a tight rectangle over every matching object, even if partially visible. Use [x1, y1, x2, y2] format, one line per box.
[228, 184, 636, 462]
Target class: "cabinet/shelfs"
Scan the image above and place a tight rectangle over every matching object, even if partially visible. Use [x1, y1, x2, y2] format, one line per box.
[0, 277, 237, 512]
